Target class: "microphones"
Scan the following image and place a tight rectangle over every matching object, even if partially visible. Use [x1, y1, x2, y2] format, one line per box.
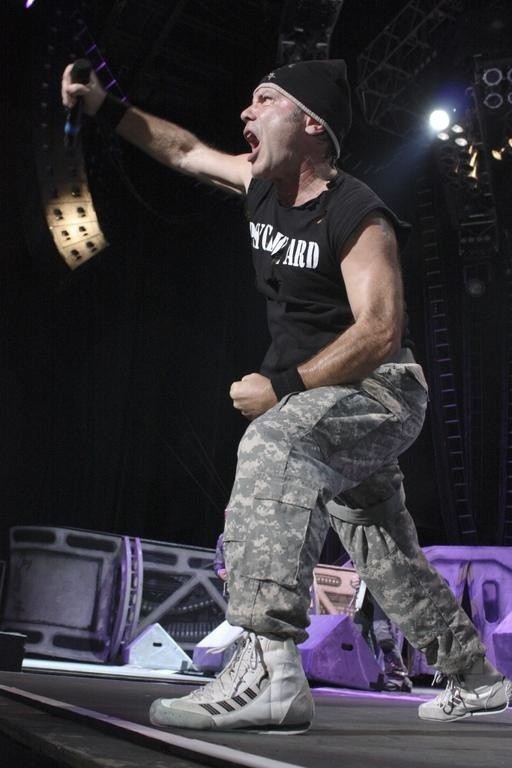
[63, 58, 94, 158]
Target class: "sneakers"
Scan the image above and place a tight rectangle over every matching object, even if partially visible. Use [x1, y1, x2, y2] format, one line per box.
[417, 670, 512, 725]
[149, 630, 315, 737]
[382, 677, 413, 693]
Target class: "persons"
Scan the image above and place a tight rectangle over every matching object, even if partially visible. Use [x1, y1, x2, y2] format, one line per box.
[62, 58, 510, 739]
[213, 504, 229, 597]
[350, 573, 416, 694]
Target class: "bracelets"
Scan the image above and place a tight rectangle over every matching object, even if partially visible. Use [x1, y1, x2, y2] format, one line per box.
[92, 94, 132, 135]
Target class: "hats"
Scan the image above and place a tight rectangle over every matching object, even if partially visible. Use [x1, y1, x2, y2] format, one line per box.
[252, 60, 352, 160]
